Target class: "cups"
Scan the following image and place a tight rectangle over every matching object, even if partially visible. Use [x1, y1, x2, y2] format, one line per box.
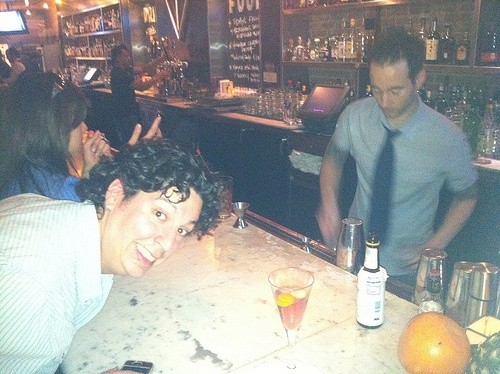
[244, 90, 304, 124]
[447, 260, 499, 328]
[414, 248, 448, 313]
[335, 218, 364, 275]
[215, 175, 234, 220]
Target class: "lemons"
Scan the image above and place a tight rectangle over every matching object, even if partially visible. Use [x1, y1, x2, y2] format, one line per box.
[277, 294, 295, 306]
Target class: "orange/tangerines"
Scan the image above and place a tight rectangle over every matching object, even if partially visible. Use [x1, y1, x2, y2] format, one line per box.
[398, 312, 471, 374]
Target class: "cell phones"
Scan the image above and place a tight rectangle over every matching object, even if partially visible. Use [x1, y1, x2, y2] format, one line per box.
[120, 360, 154, 374]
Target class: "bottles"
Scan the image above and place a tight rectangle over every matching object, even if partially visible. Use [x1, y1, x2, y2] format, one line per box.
[328, 76, 372, 103]
[59, 8, 122, 78]
[385, 16, 471, 65]
[476, 30, 500, 66]
[287, 77, 309, 94]
[418, 77, 500, 159]
[356, 233, 388, 329]
[281, 16, 373, 63]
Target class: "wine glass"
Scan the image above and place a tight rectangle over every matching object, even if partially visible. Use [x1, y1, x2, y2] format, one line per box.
[180, 78, 208, 103]
[267, 267, 315, 374]
[231, 202, 250, 229]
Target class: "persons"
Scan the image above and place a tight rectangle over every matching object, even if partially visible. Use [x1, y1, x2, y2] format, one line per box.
[318, 29, 479, 286]
[0, 44, 170, 203]
[0, 138, 231, 374]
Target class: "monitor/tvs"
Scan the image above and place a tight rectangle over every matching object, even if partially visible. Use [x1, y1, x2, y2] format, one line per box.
[84, 67, 100, 82]
[295, 83, 350, 122]
[0, 10, 29, 37]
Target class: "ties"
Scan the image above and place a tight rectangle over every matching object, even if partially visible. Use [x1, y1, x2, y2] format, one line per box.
[366, 128, 401, 244]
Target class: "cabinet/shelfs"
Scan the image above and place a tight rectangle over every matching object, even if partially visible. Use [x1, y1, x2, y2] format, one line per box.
[56, 0, 124, 82]
[137, 0, 500, 264]
